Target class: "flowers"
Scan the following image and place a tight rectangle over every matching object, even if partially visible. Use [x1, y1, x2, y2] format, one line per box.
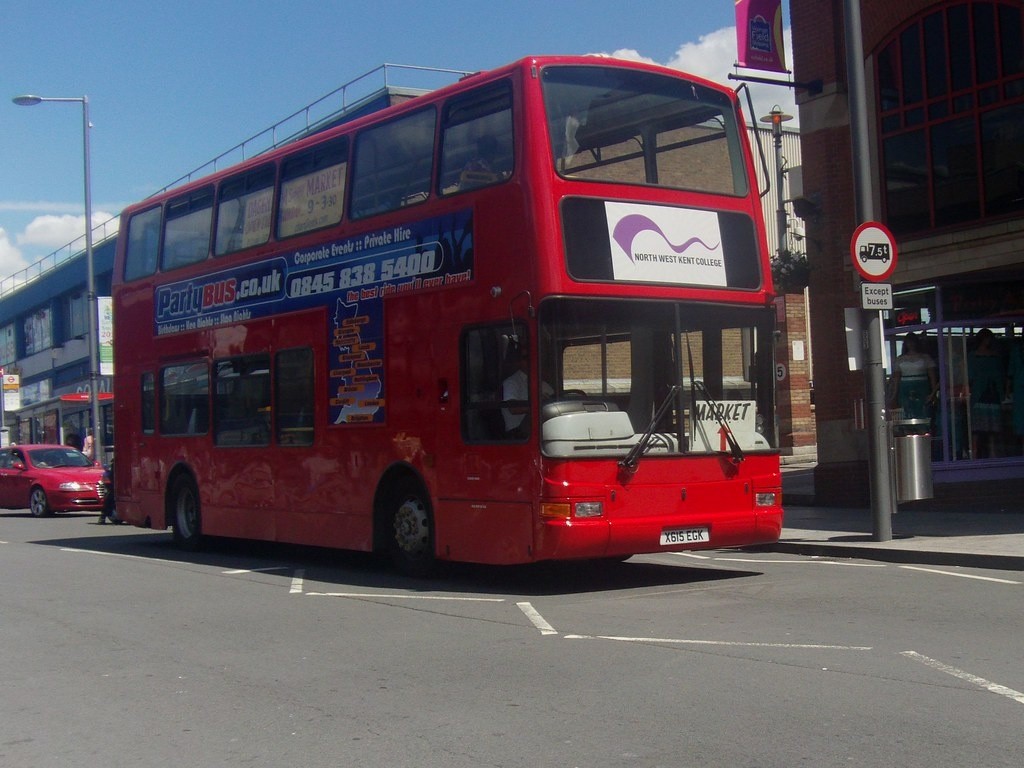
[766, 247, 809, 288]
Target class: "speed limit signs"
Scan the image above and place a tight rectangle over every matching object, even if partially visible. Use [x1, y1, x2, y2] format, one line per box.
[775, 361, 787, 382]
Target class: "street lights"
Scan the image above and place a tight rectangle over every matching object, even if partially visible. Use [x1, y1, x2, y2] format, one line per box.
[759, 104, 805, 262]
[11, 93, 107, 466]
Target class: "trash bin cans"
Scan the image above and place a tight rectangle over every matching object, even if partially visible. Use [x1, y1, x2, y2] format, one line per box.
[892, 418, 934, 509]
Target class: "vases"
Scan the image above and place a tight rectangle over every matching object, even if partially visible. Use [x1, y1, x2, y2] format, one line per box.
[781, 275, 804, 294]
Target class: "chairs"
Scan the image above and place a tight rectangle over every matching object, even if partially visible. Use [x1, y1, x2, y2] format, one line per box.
[143, 390, 315, 439]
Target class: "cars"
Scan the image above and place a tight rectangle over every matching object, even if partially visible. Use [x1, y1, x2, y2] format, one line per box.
[0, 443, 111, 518]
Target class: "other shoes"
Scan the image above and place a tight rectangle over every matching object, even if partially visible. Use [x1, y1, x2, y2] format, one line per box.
[98, 518, 105, 525]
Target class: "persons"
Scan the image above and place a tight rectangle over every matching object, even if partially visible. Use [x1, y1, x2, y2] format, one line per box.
[82, 428, 94, 460]
[963, 330, 1007, 458]
[98, 458, 115, 524]
[1006, 333, 1024, 438]
[888, 333, 940, 419]
[11, 442, 16, 446]
[459, 134, 502, 190]
[501, 343, 553, 438]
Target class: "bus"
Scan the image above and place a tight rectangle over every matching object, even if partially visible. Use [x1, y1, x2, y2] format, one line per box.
[112, 54, 788, 584]
